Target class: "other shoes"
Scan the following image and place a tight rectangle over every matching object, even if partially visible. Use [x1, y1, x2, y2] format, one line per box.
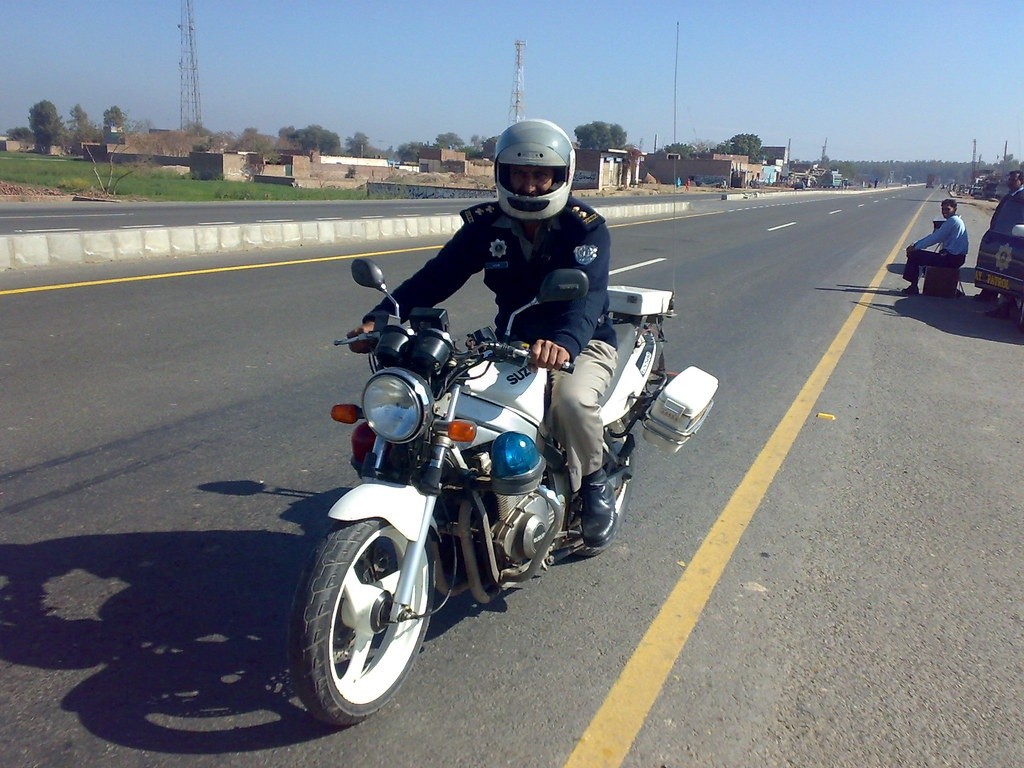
[581, 466, 616, 547]
[902, 286, 919, 295]
[974, 291, 997, 301]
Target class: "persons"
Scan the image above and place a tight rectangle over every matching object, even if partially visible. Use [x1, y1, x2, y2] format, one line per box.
[901, 199, 969, 293]
[974, 170, 1024, 320]
[349, 119, 618, 545]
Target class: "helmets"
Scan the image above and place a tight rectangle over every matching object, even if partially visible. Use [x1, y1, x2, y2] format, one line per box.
[494, 118, 576, 220]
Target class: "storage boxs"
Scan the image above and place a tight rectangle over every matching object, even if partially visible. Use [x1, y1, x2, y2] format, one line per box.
[643, 365, 719, 453]
[923, 266, 962, 297]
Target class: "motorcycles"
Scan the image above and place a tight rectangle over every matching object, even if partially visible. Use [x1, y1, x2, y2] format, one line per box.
[285, 254, 721, 728]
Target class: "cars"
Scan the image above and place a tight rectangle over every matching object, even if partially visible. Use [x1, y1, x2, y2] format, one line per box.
[974, 186, 1024, 330]
